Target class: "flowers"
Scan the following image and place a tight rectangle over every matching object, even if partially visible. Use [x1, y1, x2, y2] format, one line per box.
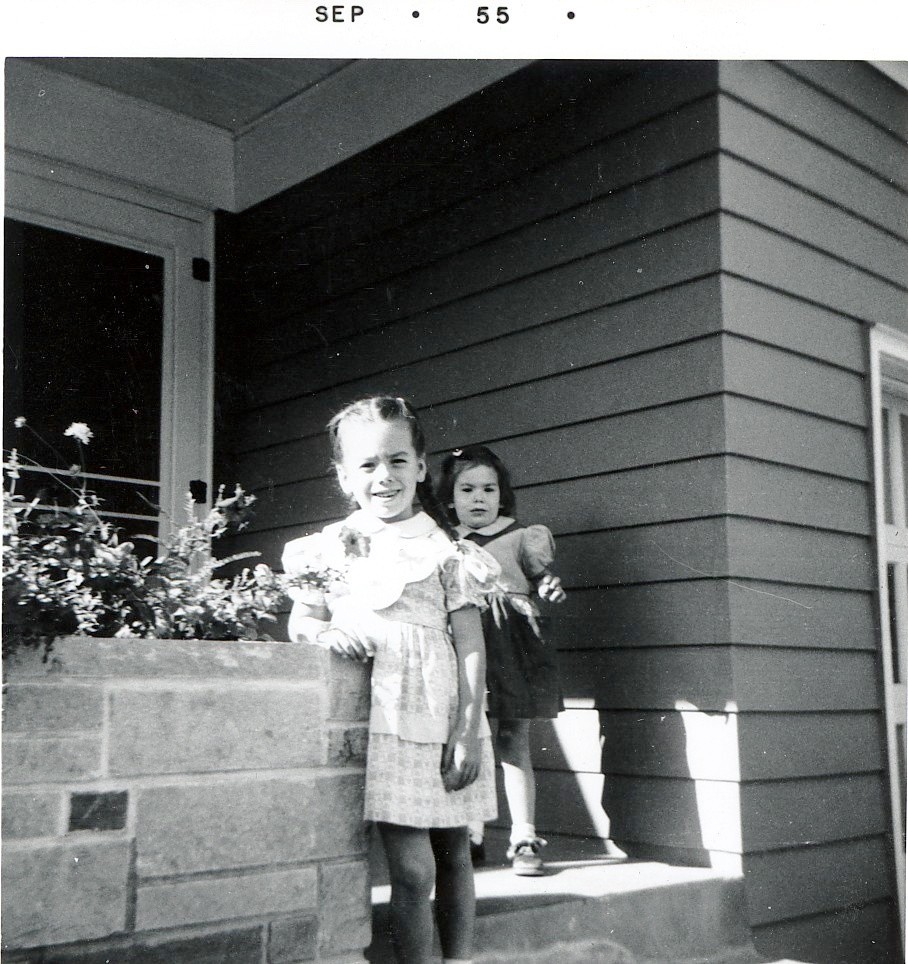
[0, 415, 375, 674]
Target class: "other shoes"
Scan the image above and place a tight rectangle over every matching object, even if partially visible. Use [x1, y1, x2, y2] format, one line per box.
[506, 841, 544, 877]
[470, 837, 486, 867]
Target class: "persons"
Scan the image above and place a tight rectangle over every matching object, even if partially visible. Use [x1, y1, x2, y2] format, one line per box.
[435, 443, 567, 876]
[280, 396, 500, 963]
